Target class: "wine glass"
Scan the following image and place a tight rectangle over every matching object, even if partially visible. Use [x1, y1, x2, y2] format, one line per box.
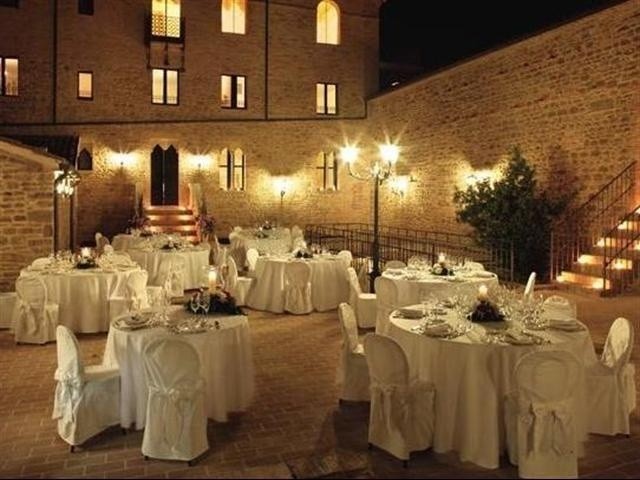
[407, 253, 473, 275]
[415, 280, 543, 335]
[56, 248, 81, 274]
[310, 242, 330, 255]
[190, 292, 210, 323]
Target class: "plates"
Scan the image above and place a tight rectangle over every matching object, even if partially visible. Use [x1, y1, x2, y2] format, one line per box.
[396, 294, 582, 344]
[112, 310, 213, 334]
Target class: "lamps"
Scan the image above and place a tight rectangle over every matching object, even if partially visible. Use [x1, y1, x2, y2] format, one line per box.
[275, 175, 294, 203]
[190, 154, 214, 176]
[394, 176, 414, 199]
[113, 151, 133, 176]
[471, 167, 493, 193]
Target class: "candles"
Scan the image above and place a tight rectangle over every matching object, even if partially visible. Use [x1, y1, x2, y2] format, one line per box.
[480, 285, 490, 300]
[438, 252, 446, 264]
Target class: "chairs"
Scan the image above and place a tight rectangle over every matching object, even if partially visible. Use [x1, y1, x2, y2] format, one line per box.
[374, 278, 398, 332]
[343, 267, 376, 328]
[141, 340, 210, 466]
[338, 303, 371, 405]
[51, 325, 120, 453]
[504, 349, 580, 479]
[524, 272, 537, 297]
[581, 317, 636, 439]
[543, 296, 578, 321]
[363, 334, 437, 469]
